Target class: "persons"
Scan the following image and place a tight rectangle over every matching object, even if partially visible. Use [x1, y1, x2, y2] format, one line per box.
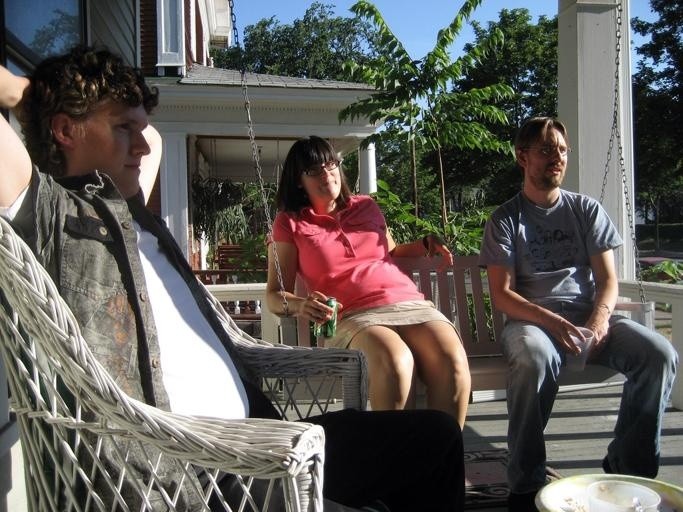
[267, 134, 472, 434]
[477, 117, 679, 509]
[0, 44, 465, 508]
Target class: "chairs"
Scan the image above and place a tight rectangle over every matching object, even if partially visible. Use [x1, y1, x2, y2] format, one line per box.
[0, 218, 368, 512]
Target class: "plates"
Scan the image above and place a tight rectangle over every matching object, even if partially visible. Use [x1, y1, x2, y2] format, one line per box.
[532, 473, 682, 512]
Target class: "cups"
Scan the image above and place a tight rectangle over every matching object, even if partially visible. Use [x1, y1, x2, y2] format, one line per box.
[565, 325, 594, 370]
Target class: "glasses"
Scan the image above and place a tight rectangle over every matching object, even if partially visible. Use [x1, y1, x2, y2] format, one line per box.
[513, 144, 572, 158]
[298, 157, 346, 178]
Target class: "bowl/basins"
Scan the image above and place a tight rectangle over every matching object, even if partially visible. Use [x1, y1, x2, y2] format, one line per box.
[585, 479, 662, 511]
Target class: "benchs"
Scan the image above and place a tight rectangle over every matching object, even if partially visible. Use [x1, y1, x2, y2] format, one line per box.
[217, 244, 268, 269]
[278, 254, 656, 403]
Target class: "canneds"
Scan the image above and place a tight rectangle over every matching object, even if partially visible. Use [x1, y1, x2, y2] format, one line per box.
[313, 297, 337, 338]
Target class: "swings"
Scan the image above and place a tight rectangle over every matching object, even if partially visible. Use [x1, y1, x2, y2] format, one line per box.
[228, 0, 655, 402]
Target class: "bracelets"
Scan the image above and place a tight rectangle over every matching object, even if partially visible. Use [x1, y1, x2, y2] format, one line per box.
[422, 236, 429, 251]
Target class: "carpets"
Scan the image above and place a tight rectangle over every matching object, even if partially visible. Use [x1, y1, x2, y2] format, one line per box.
[464, 447, 559, 512]
